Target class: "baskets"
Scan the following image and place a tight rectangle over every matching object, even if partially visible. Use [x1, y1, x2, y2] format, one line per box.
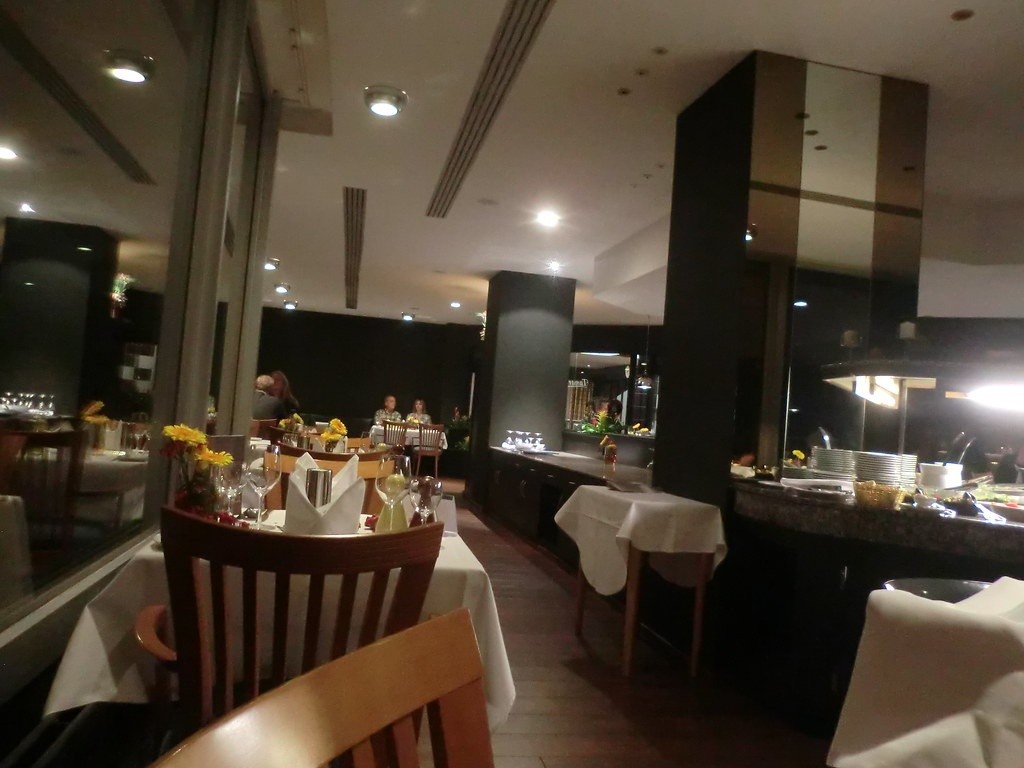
[854, 481, 906, 510]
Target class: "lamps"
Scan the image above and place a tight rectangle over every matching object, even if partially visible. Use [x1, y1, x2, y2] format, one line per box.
[637, 315, 652, 389]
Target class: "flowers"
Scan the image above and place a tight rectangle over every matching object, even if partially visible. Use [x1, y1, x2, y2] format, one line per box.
[585, 411, 649, 435]
[320, 418, 349, 451]
[161, 423, 235, 508]
[792, 449, 805, 464]
[279, 413, 304, 429]
[408, 416, 420, 424]
[80, 401, 109, 425]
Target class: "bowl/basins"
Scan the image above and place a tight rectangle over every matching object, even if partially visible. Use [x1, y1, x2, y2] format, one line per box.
[126, 450, 150, 460]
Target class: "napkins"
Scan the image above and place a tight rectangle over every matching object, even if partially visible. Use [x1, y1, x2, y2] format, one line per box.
[315, 421, 329, 433]
[104, 420, 123, 449]
[296, 452, 360, 502]
[282, 472, 367, 535]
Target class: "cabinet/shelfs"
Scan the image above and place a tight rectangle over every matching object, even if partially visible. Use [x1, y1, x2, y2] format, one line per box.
[723, 512, 1024, 739]
[485, 453, 611, 566]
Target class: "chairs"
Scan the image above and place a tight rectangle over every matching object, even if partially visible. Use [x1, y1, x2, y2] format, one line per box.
[383, 419, 445, 479]
[144, 608, 498, 768]
[0, 414, 149, 571]
[134, 506, 444, 768]
[263, 443, 406, 512]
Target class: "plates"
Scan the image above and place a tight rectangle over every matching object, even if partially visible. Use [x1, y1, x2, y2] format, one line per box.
[783, 447, 918, 490]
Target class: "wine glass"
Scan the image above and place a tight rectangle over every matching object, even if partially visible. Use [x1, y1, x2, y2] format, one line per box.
[245, 444, 281, 530]
[0, 392, 58, 417]
[210, 462, 245, 514]
[504, 430, 545, 450]
[411, 477, 443, 526]
[376, 457, 412, 531]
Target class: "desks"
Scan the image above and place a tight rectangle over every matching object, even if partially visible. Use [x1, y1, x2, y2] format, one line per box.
[370, 425, 448, 450]
[1, 495, 35, 603]
[239, 436, 270, 511]
[16, 447, 148, 525]
[556, 484, 728, 681]
[42, 506, 515, 739]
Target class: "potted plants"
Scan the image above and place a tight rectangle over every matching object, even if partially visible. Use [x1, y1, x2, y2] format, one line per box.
[445, 415, 473, 478]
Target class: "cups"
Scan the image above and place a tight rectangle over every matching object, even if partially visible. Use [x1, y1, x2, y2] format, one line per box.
[920, 464, 949, 489]
[122, 423, 136, 451]
[937, 463, 963, 490]
[299, 436, 310, 450]
[305, 468, 333, 508]
[315, 422, 329, 433]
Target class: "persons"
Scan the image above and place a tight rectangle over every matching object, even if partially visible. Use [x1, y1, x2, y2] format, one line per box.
[375, 396, 401, 427]
[252, 371, 298, 421]
[406, 399, 432, 425]
